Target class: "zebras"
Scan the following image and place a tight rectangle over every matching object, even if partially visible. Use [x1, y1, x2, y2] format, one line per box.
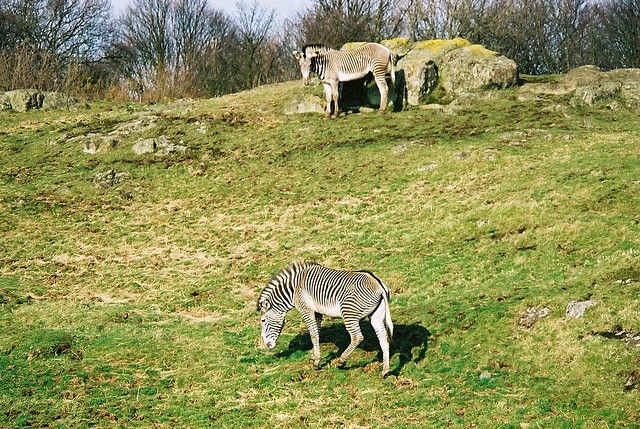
[291, 41, 396, 120]
[256, 259, 395, 379]
